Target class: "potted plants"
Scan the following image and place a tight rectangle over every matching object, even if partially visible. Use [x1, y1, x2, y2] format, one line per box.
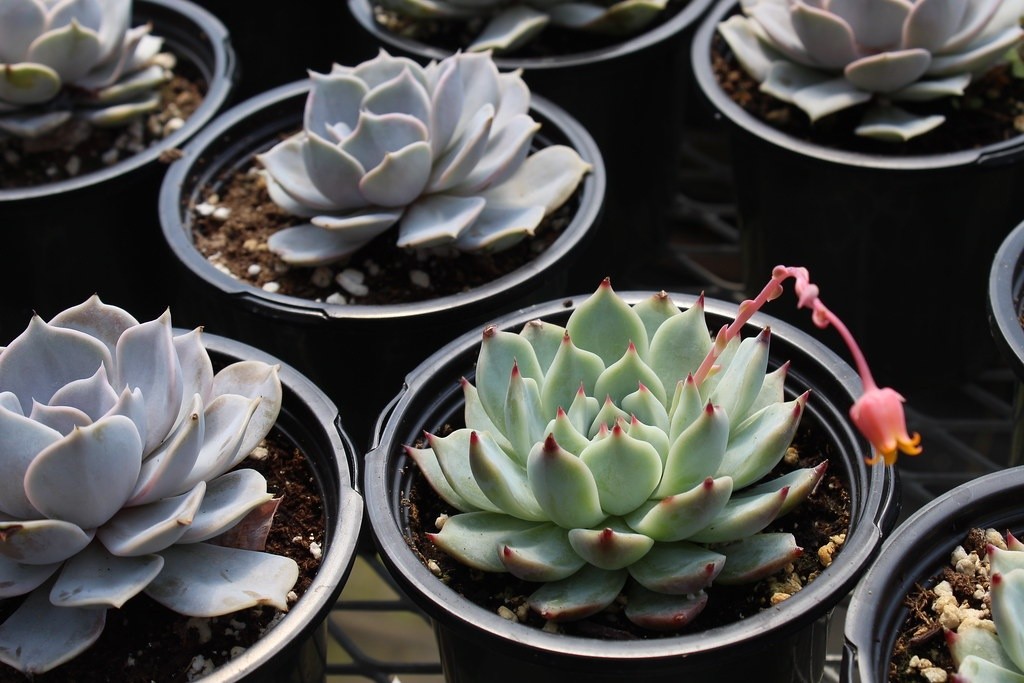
[347, 0, 717, 290]
[0, 0, 240, 347]
[159, 46, 606, 561]
[689, 0, 1024, 417]
[0, 291, 365, 683]
[364, 264, 922, 683]
[840, 465, 1024, 683]
